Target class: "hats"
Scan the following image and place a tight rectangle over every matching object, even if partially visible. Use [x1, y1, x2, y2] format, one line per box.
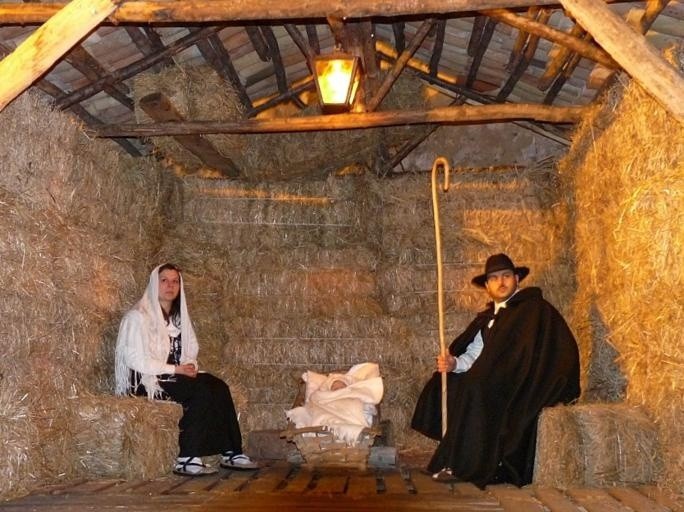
[470, 252, 531, 290]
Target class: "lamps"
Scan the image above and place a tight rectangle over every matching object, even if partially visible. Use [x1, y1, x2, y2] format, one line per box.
[308, 44, 365, 111]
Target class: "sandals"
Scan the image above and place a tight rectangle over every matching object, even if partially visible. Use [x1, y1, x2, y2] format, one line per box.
[171, 456, 220, 477]
[219, 452, 261, 471]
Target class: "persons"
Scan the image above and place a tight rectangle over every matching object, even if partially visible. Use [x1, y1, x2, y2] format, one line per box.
[114, 262, 259, 477]
[319, 372, 356, 392]
[409, 253, 581, 490]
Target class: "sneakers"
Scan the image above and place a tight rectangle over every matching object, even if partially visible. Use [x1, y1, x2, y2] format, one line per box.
[430, 466, 462, 483]
